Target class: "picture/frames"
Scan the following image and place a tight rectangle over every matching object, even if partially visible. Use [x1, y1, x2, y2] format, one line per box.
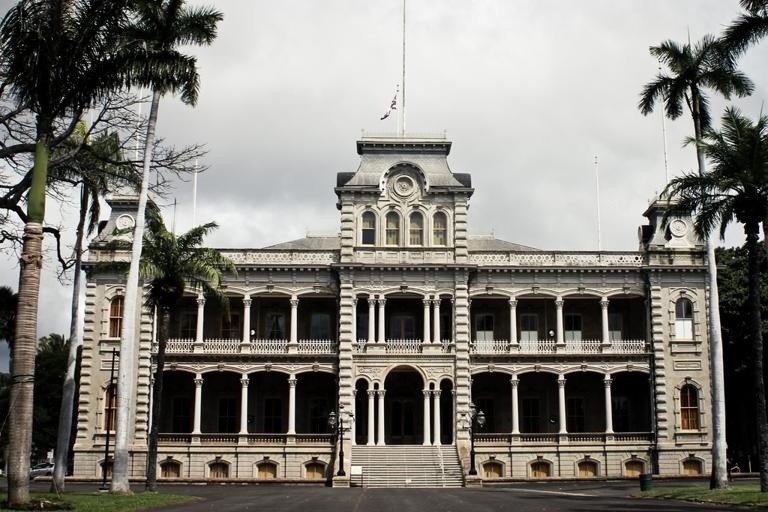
[350, 466, 363, 487]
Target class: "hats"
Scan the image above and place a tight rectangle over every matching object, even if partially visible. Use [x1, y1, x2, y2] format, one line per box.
[30, 462, 55, 479]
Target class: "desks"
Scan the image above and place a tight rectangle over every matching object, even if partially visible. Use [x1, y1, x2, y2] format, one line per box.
[468, 410, 485, 477]
[328, 411, 345, 477]
